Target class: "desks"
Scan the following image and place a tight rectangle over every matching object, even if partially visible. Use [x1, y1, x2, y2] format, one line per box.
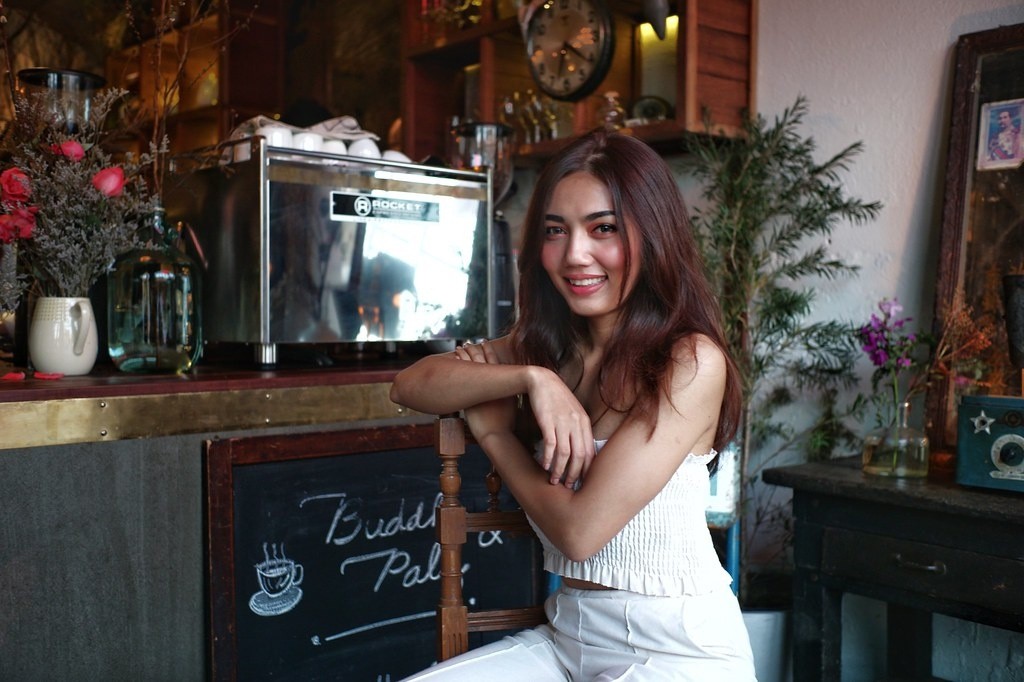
[0, 353, 440, 682]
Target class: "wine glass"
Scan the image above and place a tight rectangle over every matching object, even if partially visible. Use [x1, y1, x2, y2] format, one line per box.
[496, 86, 568, 145]
[443, 104, 480, 172]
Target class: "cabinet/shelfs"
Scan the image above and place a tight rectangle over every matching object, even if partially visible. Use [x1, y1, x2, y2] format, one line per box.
[103, 0, 286, 192]
[763, 451, 1024, 682]
[399, 0, 759, 168]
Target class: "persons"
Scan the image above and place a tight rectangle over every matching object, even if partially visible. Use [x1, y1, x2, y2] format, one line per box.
[389, 122, 762, 682]
[985, 110, 1021, 162]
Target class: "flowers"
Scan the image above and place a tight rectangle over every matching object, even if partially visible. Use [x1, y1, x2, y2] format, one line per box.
[855, 298, 992, 470]
[0, 83, 166, 315]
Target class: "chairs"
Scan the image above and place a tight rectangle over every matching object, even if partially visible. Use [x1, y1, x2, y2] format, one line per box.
[434, 411, 549, 662]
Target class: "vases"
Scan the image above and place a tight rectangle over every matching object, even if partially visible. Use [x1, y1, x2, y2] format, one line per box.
[106, 200, 204, 372]
[30, 296, 99, 375]
[862, 400, 930, 477]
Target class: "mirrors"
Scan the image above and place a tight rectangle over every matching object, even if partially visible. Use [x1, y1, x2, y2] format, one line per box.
[921, 23, 1024, 469]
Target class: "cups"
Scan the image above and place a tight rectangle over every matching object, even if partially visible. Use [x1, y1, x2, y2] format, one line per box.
[214, 125, 415, 176]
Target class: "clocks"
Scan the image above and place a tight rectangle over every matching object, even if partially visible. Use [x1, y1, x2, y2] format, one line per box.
[526, 0, 616, 102]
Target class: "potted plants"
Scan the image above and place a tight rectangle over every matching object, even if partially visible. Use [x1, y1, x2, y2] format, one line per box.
[673, 96, 884, 682]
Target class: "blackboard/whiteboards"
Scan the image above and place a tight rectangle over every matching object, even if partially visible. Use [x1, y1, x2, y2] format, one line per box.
[202, 417, 551, 682]
